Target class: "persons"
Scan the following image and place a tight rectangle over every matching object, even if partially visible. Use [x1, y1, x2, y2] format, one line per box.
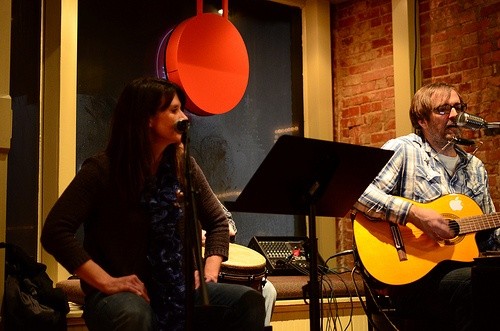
[38, 78, 266, 331]
[351, 82, 500, 331]
[5, 196, 276, 331]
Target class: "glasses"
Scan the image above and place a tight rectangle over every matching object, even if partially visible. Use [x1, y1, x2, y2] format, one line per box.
[428, 103, 467, 115]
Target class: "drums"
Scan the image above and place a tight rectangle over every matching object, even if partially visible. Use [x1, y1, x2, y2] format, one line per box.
[152, 13, 250, 117]
[198, 243, 269, 290]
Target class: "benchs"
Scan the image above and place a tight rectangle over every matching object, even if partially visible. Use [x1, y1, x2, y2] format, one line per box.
[57, 272, 368, 331]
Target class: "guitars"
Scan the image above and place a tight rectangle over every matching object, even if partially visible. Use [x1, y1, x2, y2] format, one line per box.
[352, 192, 500, 286]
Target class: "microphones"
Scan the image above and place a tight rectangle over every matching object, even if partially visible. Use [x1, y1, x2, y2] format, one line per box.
[457, 112, 500, 136]
[174, 119, 189, 133]
